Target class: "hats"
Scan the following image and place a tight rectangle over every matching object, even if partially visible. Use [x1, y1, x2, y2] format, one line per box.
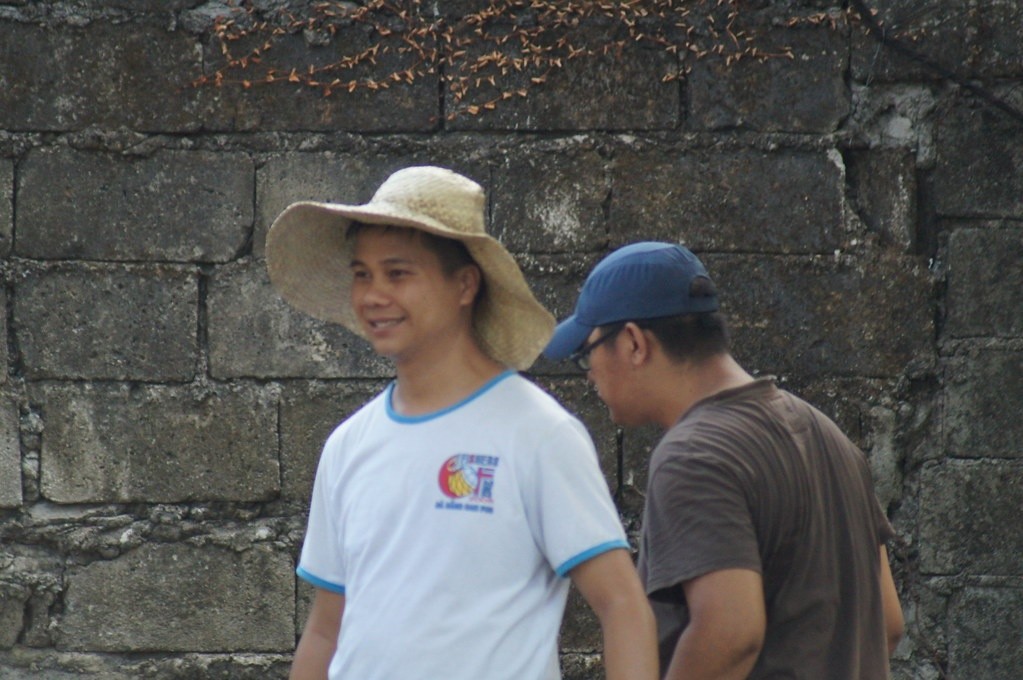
[267, 165, 556, 373]
[541, 239, 726, 358]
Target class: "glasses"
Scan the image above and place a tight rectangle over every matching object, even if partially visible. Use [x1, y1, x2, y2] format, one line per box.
[572, 326, 631, 370]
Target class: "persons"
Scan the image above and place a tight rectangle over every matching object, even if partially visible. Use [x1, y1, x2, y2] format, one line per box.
[539, 242, 910, 680]
[286, 165, 660, 680]
